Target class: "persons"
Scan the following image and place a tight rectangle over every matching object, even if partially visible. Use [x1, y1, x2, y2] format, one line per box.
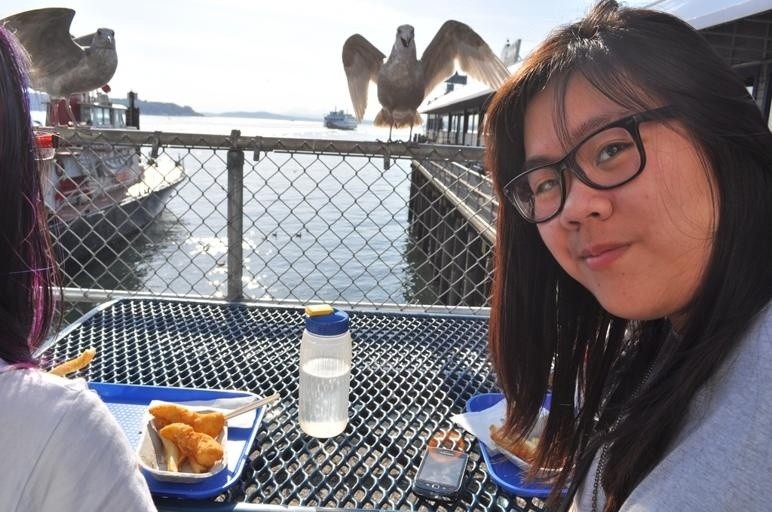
[484, 0, 772, 512]
[0, 26, 162, 512]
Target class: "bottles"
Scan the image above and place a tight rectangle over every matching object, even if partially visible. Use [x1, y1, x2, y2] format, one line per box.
[299, 304, 354, 440]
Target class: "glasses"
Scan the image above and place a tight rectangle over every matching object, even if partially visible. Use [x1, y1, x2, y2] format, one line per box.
[503, 106, 676, 224]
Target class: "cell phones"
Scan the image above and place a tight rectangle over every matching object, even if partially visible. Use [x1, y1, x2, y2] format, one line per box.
[412, 446, 469, 503]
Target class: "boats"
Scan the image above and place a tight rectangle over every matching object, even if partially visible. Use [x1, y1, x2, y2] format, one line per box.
[28, 88, 186, 265]
[324, 110, 358, 130]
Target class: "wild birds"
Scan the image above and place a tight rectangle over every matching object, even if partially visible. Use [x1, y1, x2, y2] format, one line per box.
[0, 7, 117, 134]
[341, 19, 513, 146]
